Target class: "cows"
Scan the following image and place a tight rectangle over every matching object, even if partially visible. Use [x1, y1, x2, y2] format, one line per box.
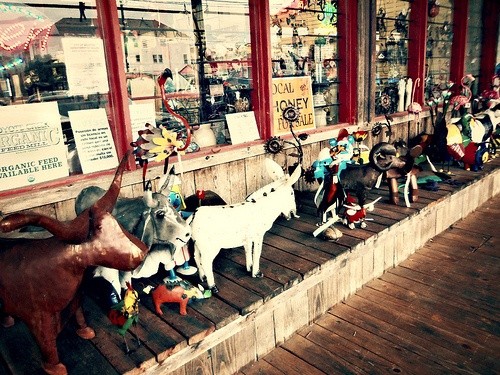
[0, 149, 149, 375]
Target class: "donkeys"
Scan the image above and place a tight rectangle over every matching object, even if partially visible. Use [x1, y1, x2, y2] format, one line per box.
[74, 165, 193, 304]
[186, 157, 302, 294]
